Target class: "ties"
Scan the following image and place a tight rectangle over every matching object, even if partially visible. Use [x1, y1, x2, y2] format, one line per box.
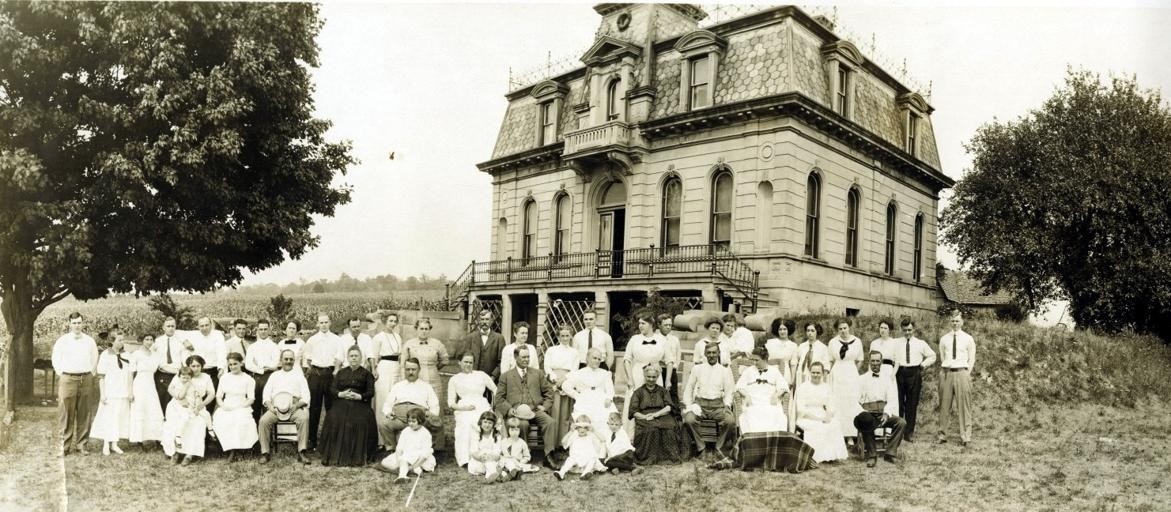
[953, 335, 956, 359]
[906, 339, 909, 363]
[354, 337, 357, 345]
[241, 341, 247, 356]
[167, 338, 172, 364]
[611, 432, 615, 441]
[588, 330, 592, 348]
[522, 369, 526, 374]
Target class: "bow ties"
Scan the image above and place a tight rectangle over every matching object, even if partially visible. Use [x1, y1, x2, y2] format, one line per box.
[285, 340, 296, 344]
[642, 340, 656, 345]
[480, 331, 489, 336]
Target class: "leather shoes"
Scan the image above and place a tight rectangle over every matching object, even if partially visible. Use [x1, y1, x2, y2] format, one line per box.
[543, 455, 560, 470]
[866, 457, 876, 468]
[229, 451, 237, 463]
[298, 453, 311, 464]
[259, 454, 269, 465]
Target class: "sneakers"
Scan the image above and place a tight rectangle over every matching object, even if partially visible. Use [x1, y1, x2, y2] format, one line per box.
[553, 471, 564, 480]
[580, 472, 593, 480]
[611, 468, 619, 475]
[392, 476, 409, 484]
[488, 472, 500, 484]
[112, 445, 123, 455]
[77, 448, 89, 456]
[413, 466, 424, 478]
[632, 466, 644, 476]
[170, 455, 178, 466]
[513, 404, 536, 420]
[181, 456, 191, 467]
[510, 466, 520, 477]
[208, 432, 218, 442]
[102, 445, 110, 456]
[57, 450, 69, 457]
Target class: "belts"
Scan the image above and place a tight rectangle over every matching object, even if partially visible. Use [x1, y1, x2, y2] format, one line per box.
[63, 372, 91, 376]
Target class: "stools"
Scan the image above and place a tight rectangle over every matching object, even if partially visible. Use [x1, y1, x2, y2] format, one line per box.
[275, 423, 299, 456]
[527, 423, 545, 450]
[700, 418, 725, 446]
[857, 426, 894, 460]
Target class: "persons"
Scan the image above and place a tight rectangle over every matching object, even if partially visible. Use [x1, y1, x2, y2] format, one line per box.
[50, 308, 450, 485]
[447, 309, 974, 486]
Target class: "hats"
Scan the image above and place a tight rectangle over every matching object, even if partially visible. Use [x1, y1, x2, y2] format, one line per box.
[272, 391, 292, 420]
[571, 414, 593, 429]
